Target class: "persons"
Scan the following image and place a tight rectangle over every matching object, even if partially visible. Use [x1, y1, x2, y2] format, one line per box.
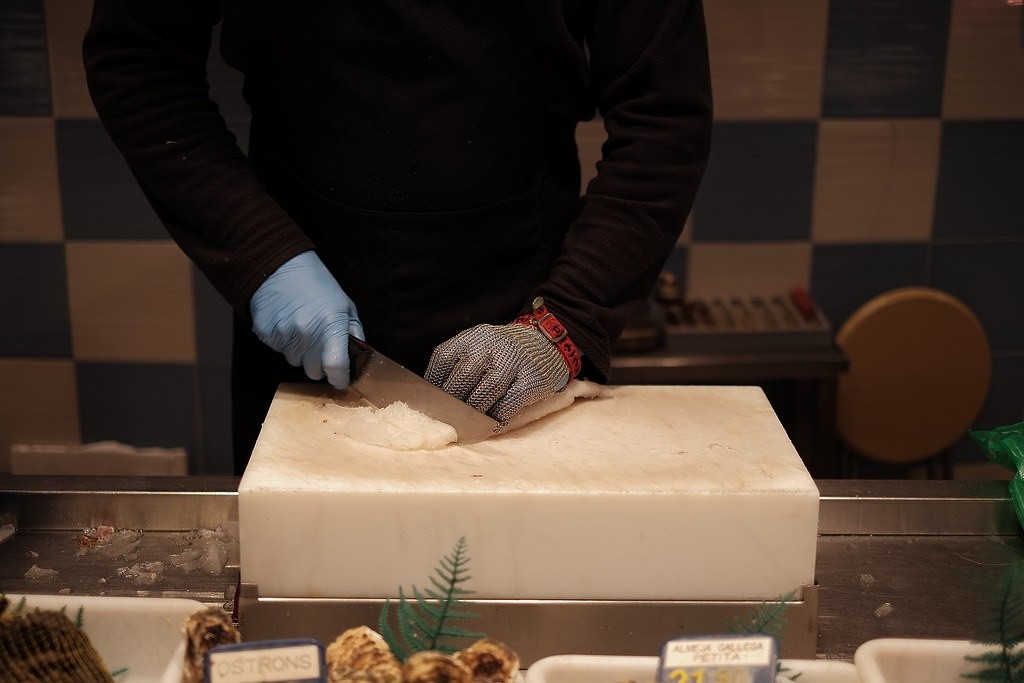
[81, 0, 713, 478]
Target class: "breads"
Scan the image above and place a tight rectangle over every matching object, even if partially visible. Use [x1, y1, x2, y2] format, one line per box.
[184, 607, 524, 683]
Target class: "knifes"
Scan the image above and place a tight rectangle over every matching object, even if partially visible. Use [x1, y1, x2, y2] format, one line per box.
[348, 334, 499, 446]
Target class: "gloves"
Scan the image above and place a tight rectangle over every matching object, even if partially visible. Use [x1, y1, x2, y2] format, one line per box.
[420, 322, 570, 422]
[249, 251, 367, 389]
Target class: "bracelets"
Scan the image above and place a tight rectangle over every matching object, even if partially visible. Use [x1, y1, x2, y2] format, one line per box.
[510, 297, 581, 384]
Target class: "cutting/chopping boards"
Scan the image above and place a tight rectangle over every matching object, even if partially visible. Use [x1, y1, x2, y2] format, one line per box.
[237, 381, 820, 601]
